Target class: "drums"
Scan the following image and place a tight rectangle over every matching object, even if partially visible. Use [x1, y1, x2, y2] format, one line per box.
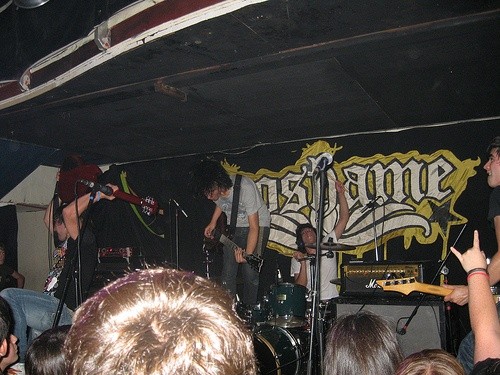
[253, 326, 303, 375]
[265, 281, 311, 328]
[244, 301, 269, 328]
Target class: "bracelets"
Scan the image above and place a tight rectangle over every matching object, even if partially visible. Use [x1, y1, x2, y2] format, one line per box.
[97, 190, 100, 201]
[466, 268, 490, 281]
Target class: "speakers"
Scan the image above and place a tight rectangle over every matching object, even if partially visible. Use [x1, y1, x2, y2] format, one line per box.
[330, 298, 450, 361]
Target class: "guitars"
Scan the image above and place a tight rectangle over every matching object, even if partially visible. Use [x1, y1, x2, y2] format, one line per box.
[206, 213, 267, 276]
[58, 166, 160, 215]
[375, 277, 500, 306]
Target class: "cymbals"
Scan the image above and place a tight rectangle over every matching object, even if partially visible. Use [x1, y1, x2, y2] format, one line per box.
[305, 242, 357, 252]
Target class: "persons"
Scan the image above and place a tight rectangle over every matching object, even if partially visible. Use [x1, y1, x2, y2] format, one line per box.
[0, 314, 19, 375]
[322, 310, 403, 375]
[24, 325, 71, 375]
[0, 246, 24, 292]
[442, 139, 500, 313]
[291, 179, 350, 311]
[0, 184, 119, 375]
[63, 268, 259, 375]
[395, 349, 465, 375]
[449, 230, 500, 375]
[190, 159, 272, 305]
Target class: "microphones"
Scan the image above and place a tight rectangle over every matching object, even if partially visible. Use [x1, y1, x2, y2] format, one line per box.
[277, 265, 283, 283]
[174, 199, 188, 217]
[399, 328, 406, 334]
[79, 180, 113, 196]
[360, 196, 380, 213]
[314, 153, 332, 171]
